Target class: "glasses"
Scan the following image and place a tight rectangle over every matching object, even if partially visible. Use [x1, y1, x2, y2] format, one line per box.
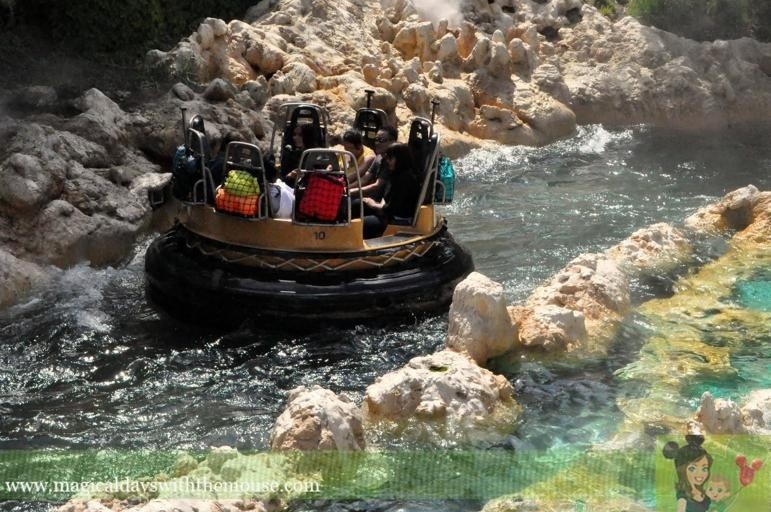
[374, 136, 386, 143]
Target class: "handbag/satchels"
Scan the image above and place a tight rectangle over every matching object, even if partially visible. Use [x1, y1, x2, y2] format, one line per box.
[437, 156, 454, 200]
[297, 173, 344, 222]
[175, 177, 205, 201]
[174, 144, 203, 179]
[224, 169, 261, 197]
[215, 189, 259, 216]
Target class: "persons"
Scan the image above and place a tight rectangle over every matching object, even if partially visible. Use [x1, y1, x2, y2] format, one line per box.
[213, 126, 422, 236]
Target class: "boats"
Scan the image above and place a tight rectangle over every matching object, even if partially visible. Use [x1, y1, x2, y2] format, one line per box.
[143, 89, 475, 343]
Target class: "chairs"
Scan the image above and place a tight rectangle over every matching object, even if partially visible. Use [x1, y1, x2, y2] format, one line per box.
[185, 105, 446, 227]
